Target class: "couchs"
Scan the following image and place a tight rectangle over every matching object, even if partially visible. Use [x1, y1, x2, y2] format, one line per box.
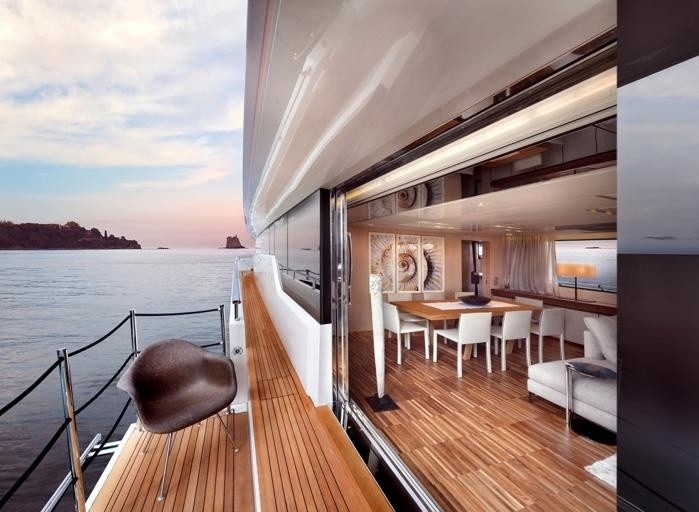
[525, 342, 618, 437]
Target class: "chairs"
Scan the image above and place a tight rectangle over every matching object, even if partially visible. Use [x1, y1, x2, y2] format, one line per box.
[117, 338, 241, 503]
[383, 291, 566, 378]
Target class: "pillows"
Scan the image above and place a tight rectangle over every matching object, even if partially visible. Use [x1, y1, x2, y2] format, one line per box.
[581, 312, 618, 364]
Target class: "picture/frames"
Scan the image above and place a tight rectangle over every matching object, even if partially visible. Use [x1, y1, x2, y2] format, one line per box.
[368, 231, 446, 294]
